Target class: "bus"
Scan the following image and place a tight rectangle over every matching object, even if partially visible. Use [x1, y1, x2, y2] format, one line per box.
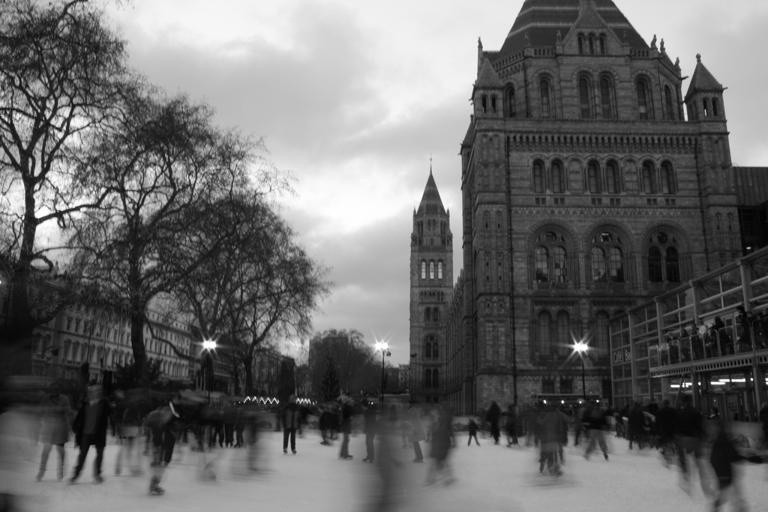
[529, 393, 601, 409]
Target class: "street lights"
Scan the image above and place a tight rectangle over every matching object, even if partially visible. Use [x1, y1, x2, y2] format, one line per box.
[573, 337, 593, 407]
[542, 399, 600, 410]
[203, 338, 217, 391]
[376, 339, 391, 407]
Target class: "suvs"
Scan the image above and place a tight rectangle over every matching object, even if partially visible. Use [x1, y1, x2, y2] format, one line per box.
[366, 394, 409, 408]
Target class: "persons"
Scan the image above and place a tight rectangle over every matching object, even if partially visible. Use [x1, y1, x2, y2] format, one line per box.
[660, 304, 768, 366]
[0, 383, 766, 511]
